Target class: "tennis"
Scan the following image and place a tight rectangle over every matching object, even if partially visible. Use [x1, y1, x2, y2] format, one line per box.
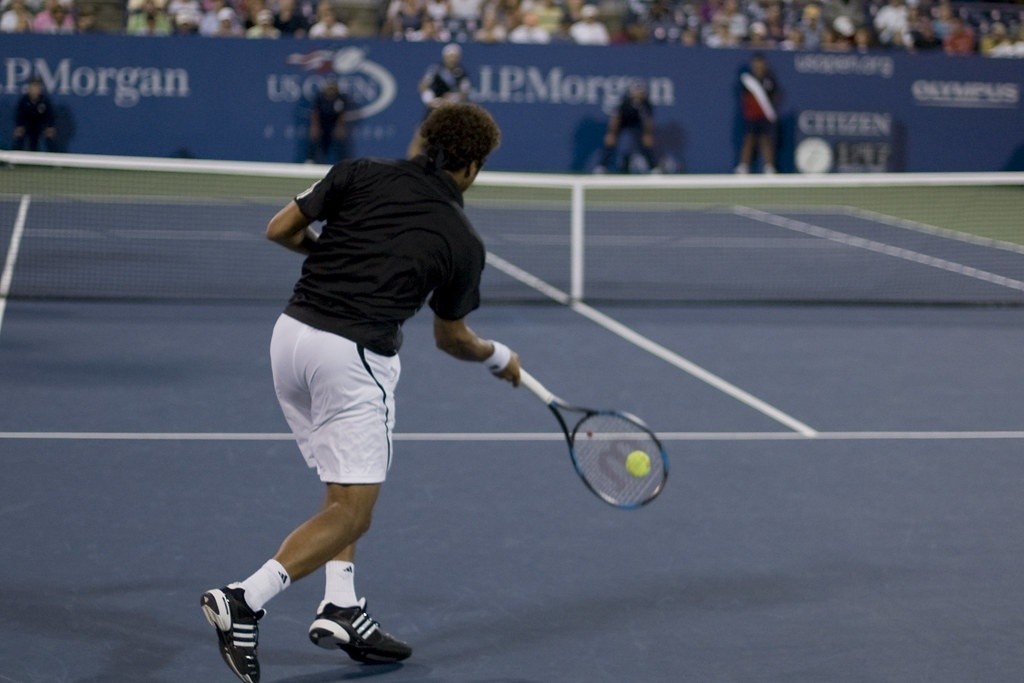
[625, 450, 652, 478]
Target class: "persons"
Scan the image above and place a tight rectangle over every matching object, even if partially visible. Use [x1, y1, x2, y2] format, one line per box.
[732, 54, 781, 174]
[300, 81, 358, 165]
[13, 77, 59, 153]
[592, 80, 656, 174]
[418, 43, 475, 110]
[200, 105, 522, 683]
[0, 0, 1024, 58]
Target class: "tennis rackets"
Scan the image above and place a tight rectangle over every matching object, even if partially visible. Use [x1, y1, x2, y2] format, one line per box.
[515, 365, 669, 509]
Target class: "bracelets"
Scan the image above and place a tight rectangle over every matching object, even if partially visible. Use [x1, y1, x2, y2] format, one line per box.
[480, 339, 512, 374]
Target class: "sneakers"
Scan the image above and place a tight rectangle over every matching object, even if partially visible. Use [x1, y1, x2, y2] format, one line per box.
[308, 597, 413, 664]
[200, 582, 268, 683]
